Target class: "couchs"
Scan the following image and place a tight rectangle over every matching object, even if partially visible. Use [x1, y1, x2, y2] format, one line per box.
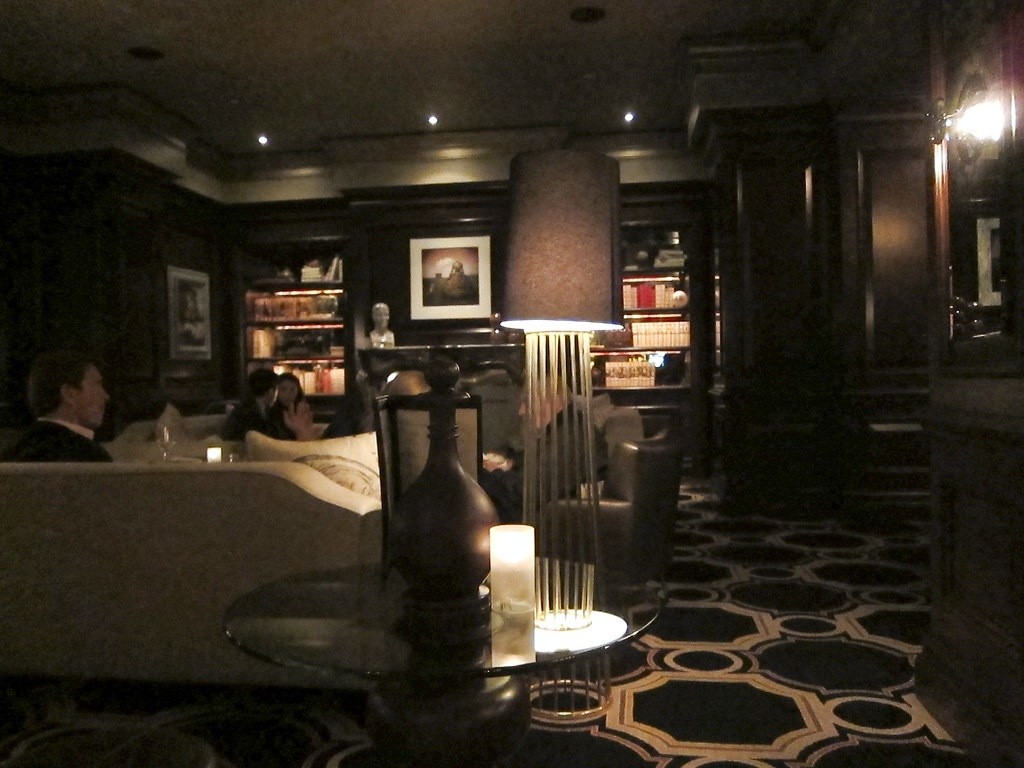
[0, 413, 381, 686]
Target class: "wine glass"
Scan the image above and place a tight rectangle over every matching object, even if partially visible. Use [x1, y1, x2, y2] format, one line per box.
[156, 421, 178, 461]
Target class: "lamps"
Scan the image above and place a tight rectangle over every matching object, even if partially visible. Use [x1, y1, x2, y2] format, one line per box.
[489, 524, 535, 614]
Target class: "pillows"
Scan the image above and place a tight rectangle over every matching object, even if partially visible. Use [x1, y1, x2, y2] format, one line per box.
[245, 429, 379, 475]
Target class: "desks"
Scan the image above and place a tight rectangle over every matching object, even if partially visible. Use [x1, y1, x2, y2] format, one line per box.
[223, 562, 662, 768]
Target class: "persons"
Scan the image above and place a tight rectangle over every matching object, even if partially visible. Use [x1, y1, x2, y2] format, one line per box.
[369, 303, 395, 348]
[0, 341, 114, 462]
[220, 366, 377, 443]
[478, 375, 608, 525]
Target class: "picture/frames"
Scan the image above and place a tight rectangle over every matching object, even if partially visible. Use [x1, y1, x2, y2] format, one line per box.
[976, 218, 1003, 307]
[167, 264, 212, 361]
[404, 231, 495, 327]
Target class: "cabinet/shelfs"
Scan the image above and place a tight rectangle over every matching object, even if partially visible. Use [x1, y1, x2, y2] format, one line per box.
[240, 235, 356, 408]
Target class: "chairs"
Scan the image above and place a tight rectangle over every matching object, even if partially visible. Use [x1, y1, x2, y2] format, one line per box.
[539, 430, 682, 602]
